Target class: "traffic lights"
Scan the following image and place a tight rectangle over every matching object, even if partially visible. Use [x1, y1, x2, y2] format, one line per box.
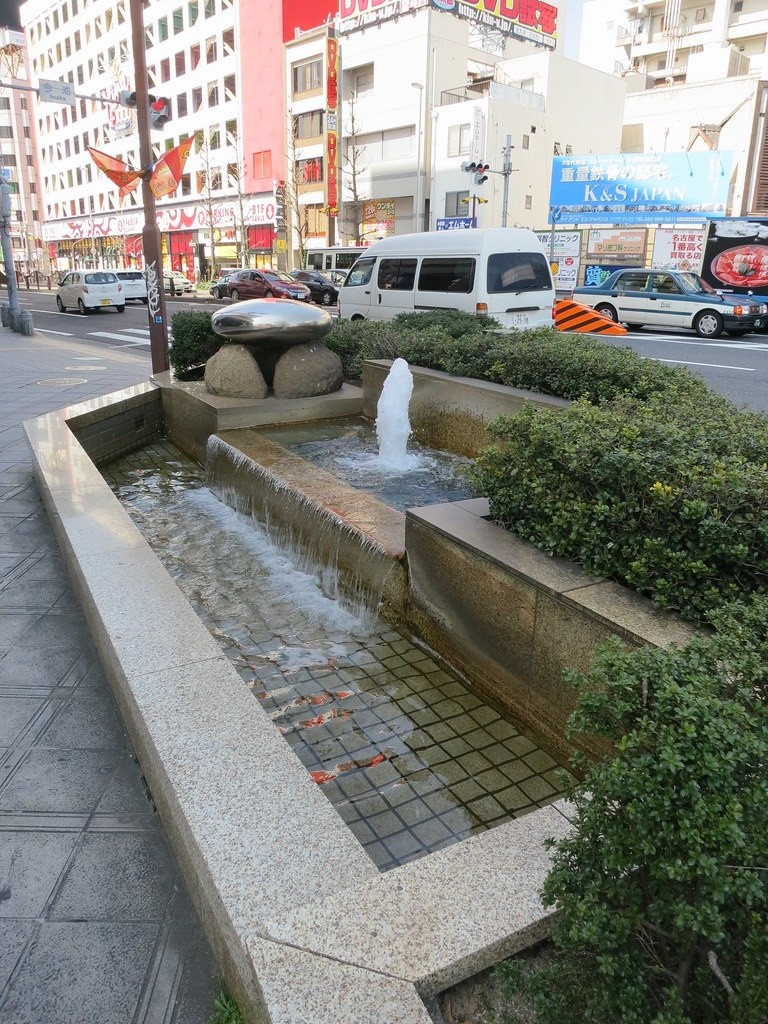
[120, 90, 169, 114]
[149, 113, 168, 131]
[474, 174, 488, 185]
[461, 161, 490, 174]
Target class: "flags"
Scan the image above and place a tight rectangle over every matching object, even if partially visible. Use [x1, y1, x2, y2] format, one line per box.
[85, 145, 145, 196]
[149, 130, 199, 198]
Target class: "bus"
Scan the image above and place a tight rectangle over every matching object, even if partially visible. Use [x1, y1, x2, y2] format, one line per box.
[305, 247, 368, 272]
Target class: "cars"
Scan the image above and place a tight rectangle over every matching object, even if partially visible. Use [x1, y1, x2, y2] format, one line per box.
[172, 270, 192, 293]
[569, 269, 768, 338]
[227, 269, 312, 303]
[209, 274, 231, 299]
[109, 269, 147, 304]
[56, 269, 125, 316]
[142, 270, 184, 296]
[288, 271, 343, 305]
[321, 269, 361, 287]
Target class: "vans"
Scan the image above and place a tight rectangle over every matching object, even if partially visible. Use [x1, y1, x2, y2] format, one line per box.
[337, 227, 556, 331]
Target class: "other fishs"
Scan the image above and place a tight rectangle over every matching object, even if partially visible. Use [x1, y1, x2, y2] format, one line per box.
[240, 645, 398, 788]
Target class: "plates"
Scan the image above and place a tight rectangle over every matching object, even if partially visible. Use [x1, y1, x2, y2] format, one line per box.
[710, 244, 768, 287]
